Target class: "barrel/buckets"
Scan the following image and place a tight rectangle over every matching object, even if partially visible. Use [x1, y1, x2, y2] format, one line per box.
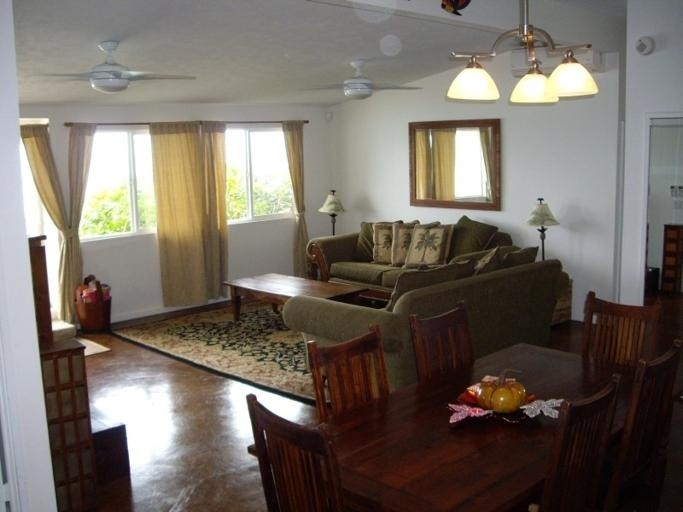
[74, 274, 111, 334]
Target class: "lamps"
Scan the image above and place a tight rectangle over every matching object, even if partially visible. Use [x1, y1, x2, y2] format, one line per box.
[525, 198, 560, 261]
[318, 190, 345, 236]
[447, 0, 600, 104]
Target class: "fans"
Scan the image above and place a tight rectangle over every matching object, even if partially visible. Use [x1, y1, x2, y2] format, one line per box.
[301, 61, 423, 98]
[42, 40, 196, 93]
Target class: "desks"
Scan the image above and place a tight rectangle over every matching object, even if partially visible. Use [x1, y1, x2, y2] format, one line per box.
[553, 278, 572, 326]
[222, 273, 369, 321]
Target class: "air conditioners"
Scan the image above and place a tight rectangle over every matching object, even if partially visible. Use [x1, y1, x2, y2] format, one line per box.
[513, 45, 602, 77]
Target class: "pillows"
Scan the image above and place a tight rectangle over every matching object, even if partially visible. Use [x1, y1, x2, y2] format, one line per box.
[356, 215, 538, 310]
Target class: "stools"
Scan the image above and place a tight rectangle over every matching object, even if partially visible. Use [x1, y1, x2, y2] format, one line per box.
[89, 407, 130, 481]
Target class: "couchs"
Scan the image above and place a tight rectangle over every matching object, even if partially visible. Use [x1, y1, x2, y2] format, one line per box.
[282, 259, 563, 383]
[306, 232, 512, 301]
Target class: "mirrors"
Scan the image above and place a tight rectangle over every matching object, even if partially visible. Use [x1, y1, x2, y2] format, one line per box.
[408, 119, 500, 211]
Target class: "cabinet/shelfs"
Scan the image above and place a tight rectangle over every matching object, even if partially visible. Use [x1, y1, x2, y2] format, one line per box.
[40, 341, 92, 512]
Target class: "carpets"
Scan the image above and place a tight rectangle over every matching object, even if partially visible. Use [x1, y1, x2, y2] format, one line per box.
[110, 305, 331, 405]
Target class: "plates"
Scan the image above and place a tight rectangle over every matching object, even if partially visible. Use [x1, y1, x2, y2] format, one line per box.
[457, 383, 536, 424]
